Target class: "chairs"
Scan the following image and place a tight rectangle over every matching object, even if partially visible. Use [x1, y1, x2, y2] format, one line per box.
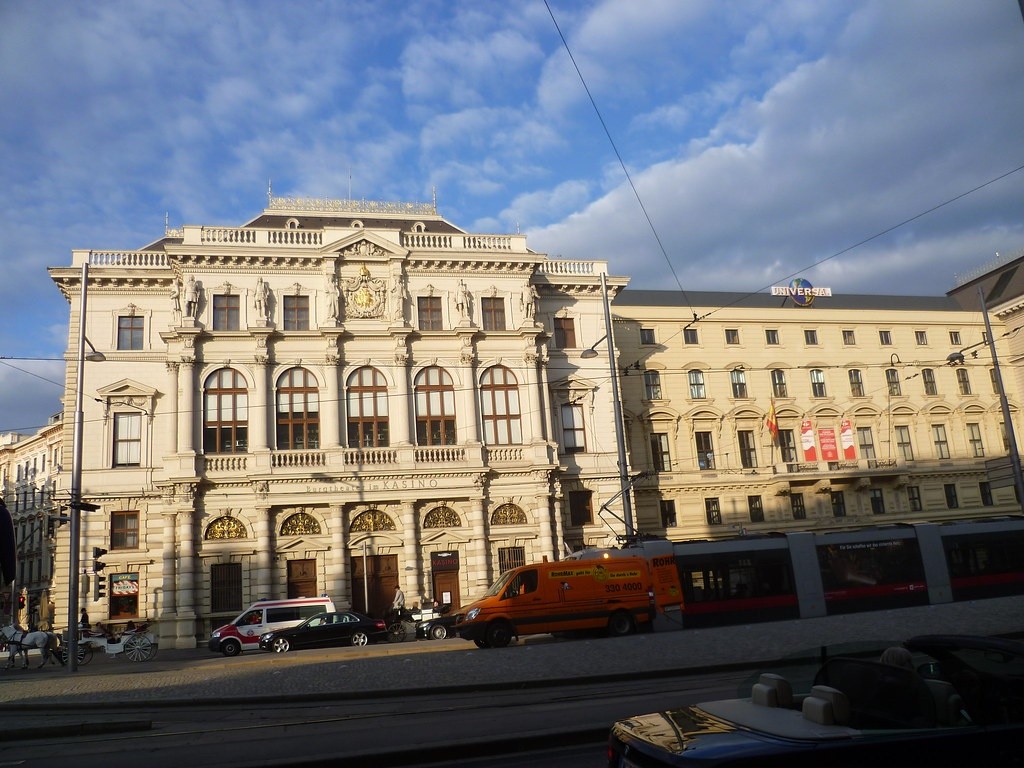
[804, 685, 853, 726]
[518, 575, 534, 594]
[752, 672, 794, 709]
[923, 679, 979, 727]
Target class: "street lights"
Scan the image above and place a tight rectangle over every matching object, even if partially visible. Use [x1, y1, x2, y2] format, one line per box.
[945, 288, 1023, 513]
[581, 270, 634, 536]
[67, 261, 105, 676]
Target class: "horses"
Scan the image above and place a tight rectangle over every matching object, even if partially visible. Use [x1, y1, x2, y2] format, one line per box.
[0, 623, 62, 669]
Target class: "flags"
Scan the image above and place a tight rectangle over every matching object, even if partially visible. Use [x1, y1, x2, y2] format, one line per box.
[766, 404, 778, 440]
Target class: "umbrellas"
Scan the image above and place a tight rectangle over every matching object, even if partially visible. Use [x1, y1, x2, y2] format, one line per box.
[37, 589, 49, 631]
[17, 588, 29, 631]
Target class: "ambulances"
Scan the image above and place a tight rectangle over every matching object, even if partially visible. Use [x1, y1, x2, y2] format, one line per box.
[208, 594, 338, 657]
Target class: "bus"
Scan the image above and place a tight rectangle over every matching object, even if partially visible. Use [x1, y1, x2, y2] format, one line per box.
[561, 474, 1023, 632]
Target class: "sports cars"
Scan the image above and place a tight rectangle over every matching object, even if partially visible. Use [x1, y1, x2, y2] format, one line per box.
[607, 630, 1023, 767]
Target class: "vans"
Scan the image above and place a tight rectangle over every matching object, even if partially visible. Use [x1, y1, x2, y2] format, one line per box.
[448, 556, 656, 649]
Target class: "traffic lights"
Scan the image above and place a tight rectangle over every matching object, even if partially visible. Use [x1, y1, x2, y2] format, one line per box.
[93, 574, 106, 602]
[93, 547, 107, 572]
[19, 596, 24, 609]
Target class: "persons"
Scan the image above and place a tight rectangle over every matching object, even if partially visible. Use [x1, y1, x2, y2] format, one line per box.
[324, 276, 340, 319]
[105, 625, 117, 643]
[881, 648, 970, 728]
[391, 276, 407, 319]
[250, 611, 262, 624]
[520, 280, 535, 318]
[80, 607, 89, 624]
[122, 620, 136, 635]
[433, 600, 440, 608]
[318, 618, 326, 626]
[453, 278, 472, 318]
[183, 273, 202, 317]
[252, 276, 271, 318]
[392, 585, 406, 608]
[412, 602, 419, 611]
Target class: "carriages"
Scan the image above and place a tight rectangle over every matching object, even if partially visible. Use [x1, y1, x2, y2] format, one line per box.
[0, 619, 160, 673]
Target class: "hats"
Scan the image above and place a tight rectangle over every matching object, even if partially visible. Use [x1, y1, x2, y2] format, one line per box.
[81, 607, 87, 612]
[393, 585, 401, 589]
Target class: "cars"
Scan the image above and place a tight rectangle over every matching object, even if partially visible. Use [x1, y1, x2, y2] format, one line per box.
[415, 616, 458, 639]
[258, 611, 389, 653]
